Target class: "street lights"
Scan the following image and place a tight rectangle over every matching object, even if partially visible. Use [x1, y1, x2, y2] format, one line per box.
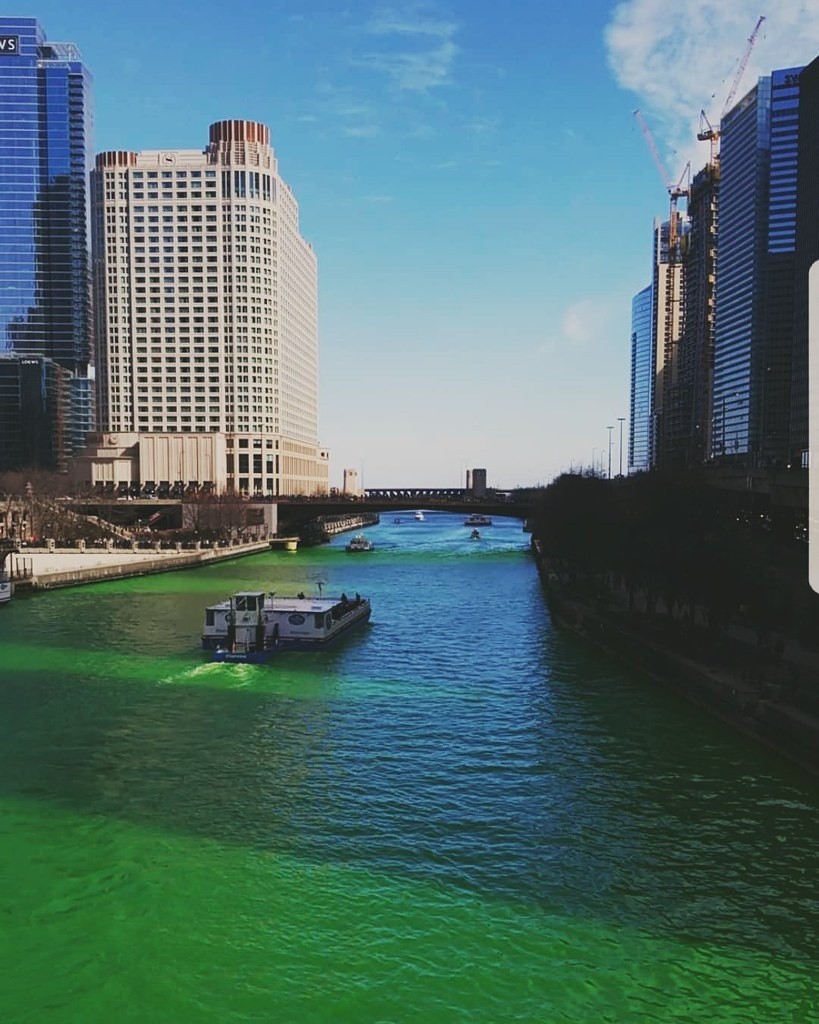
[617, 418, 626, 477]
[607, 426, 615, 479]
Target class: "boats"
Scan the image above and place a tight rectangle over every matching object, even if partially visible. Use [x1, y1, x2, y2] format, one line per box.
[198, 579, 372, 661]
[346, 533, 374, 554]
[414, 511, 424, 520]
[464, 513, 493, 526]
